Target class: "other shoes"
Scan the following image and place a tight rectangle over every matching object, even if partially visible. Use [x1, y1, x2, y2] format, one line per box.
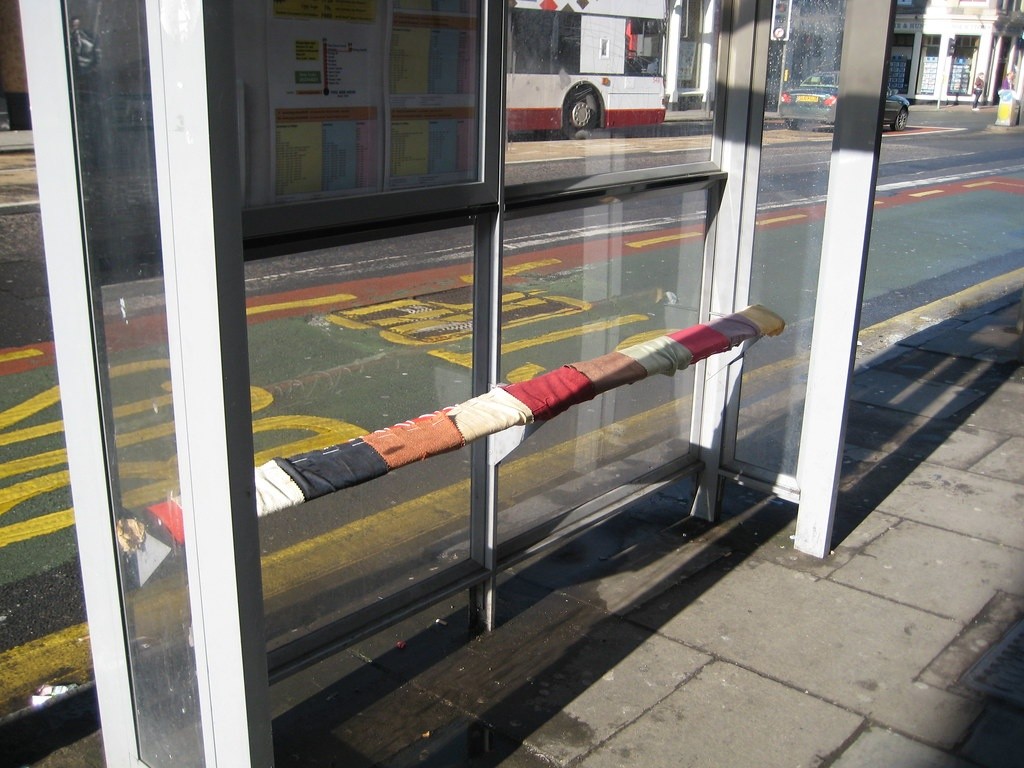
[972, 108, 979, 111]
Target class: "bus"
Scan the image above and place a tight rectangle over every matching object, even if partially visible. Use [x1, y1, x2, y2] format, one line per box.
[506, 0, 669, 140]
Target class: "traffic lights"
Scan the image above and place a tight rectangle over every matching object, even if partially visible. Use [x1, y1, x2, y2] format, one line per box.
[770, 0, 792, 41]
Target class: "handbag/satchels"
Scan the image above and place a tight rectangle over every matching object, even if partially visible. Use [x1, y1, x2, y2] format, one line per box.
[973, 89, 976, 94]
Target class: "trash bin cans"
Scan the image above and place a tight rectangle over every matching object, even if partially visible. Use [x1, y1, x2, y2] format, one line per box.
[994, 90, 1020, 125]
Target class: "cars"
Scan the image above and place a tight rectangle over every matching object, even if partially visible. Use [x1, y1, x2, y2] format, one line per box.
[778, 70, 910, 133]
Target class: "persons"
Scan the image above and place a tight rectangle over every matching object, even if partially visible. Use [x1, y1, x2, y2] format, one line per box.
[1002, 70, 1016, 91]
[971, 73, 985, 111]
